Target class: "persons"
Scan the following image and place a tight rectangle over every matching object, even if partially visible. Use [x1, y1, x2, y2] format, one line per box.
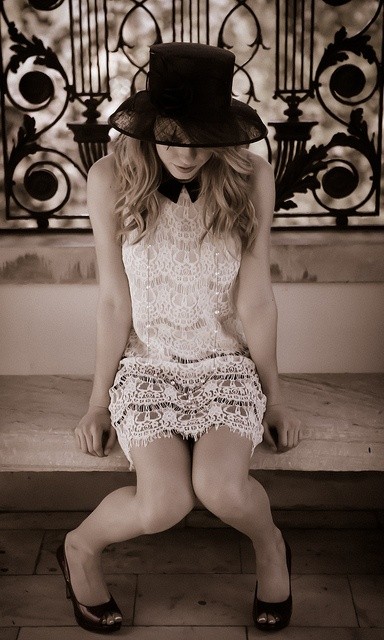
[59, 42, 302, 634]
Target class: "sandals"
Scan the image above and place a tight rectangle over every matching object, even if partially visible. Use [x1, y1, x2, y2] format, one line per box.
[56, 531, 122, 634]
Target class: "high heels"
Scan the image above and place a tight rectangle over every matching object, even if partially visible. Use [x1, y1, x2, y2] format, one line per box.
[252, 537, 292, 632]
[108, 42, 268, 148]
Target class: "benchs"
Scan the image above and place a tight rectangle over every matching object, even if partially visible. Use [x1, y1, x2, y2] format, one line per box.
[1, 374, 384, 473]
